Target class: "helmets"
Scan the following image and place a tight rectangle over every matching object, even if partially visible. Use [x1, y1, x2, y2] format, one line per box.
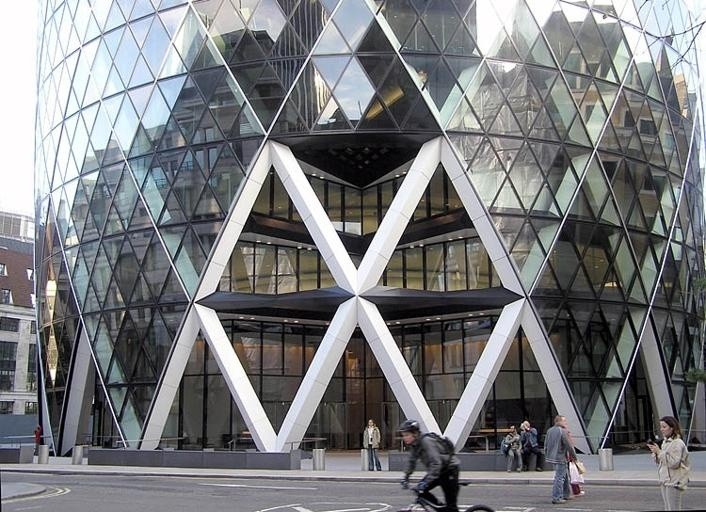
[396, 420, 419, 431]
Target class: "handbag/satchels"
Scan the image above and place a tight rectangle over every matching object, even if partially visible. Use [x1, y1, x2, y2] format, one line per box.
[569, 461, 585, 486]
[440, 436, 455, 453]
[501, 440, 511, 456]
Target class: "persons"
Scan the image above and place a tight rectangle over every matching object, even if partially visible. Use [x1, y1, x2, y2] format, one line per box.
[34, 426, 42, 456]
[504, 421, 542, 472]
[363, 419, 381, 471]
[399, 420, 460, 512]
[646, 416, 689, 511]
[544, 415, 585, 504]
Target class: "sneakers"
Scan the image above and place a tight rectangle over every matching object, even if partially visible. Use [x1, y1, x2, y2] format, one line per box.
[506, 469, 511, 473]
[566, 494, 575, 501]
[535, 467, 544, 470]
[574, 491, 585, 497]
[553, 498, 566, 503]
[517, 466, 529, 472]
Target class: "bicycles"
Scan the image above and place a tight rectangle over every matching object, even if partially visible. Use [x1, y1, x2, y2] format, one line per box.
[396, 481, 495, 512]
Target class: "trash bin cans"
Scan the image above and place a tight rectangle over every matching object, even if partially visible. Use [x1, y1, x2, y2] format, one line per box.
[313, 449, 325, 471]
[598, 448, 614, 471]
[361, 449, 370, 471]
[72, 446, 83, 464]
[39, 445, 49, 464]
